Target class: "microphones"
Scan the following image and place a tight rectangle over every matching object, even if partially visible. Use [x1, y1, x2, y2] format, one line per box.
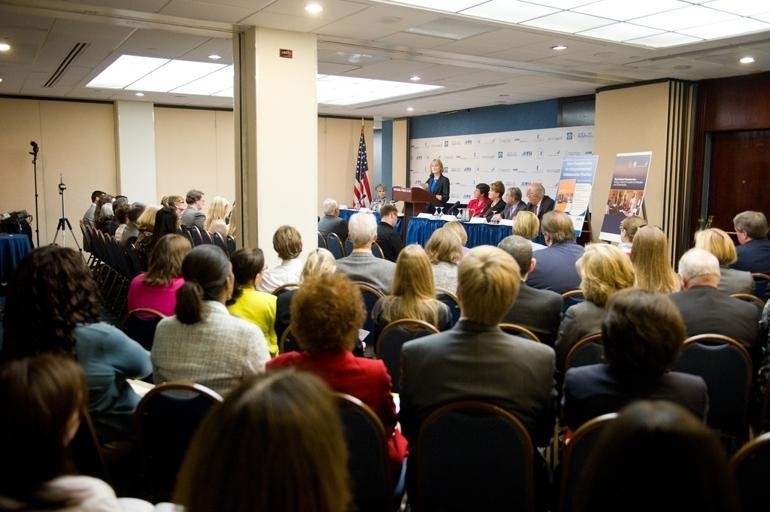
[426, 173, 434, 183]
[359, 195, 366, 201]
[449, 202, 460, 210]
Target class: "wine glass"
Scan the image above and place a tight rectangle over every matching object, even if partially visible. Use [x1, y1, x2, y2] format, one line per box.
[490, 210, 497, 224]
[434, 206, 444, 217]
[456, 207, 462, 219]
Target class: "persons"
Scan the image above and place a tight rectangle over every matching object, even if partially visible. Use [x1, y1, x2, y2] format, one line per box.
[0, 191, 770, 512]
[372, 184, 390, 212]
[467, 180, 556, 221]
[423, 159, 450, 216]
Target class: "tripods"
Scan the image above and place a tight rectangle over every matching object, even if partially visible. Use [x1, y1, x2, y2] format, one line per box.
[53, 183, 82, 252]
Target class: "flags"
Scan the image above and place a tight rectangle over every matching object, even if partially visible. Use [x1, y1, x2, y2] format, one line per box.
[354, 127, 372, 207]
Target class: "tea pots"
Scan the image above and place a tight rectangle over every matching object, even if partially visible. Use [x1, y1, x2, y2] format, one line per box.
[462, 208, 474, 221]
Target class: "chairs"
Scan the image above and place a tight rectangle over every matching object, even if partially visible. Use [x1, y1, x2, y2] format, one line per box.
[2, 205, 770, 511]
